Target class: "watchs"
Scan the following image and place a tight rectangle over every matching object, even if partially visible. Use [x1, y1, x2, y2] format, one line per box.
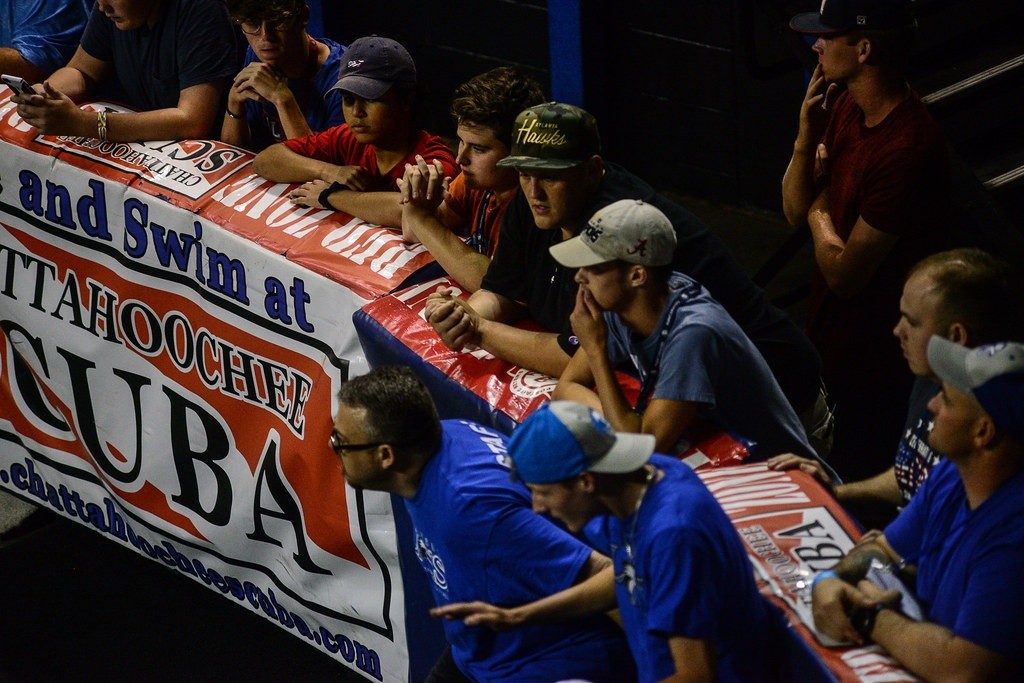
[850, 601, 894, 643]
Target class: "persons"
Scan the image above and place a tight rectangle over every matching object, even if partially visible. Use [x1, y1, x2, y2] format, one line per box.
[766, 248, 1023, 547]
[396, 67, 836, 460]
[329, 365, 634, 683]
[0, 1, 222, 148]
[550, 197, 845, 485]
[812, 335, 1024, 683]
[221, 0, 461, 227]
[429, 400, 834, 683]
[783, 1, 947, 529]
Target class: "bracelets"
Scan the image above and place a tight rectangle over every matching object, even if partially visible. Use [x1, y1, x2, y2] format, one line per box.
[811, 569, 841, 591]
[97, 99, 109, 146]
[224, 106, 248, 118]
[318, 182, 353, 212]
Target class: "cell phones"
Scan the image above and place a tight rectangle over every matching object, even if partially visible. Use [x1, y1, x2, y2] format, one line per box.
[0, 74, 37, 96]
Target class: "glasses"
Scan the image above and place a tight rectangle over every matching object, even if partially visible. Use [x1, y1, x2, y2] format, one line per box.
[330, 429, 406, 455]
[237, 8, 301, 34]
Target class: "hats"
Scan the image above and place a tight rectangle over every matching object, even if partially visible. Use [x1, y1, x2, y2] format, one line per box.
[789, 0, 913, 34]
[549, 199, 677, 268]
[506, 401, 656, 485]
[496, 101, 601, 169]
[926, 334, 1024, 438]
[323, 34, 416, 102]
